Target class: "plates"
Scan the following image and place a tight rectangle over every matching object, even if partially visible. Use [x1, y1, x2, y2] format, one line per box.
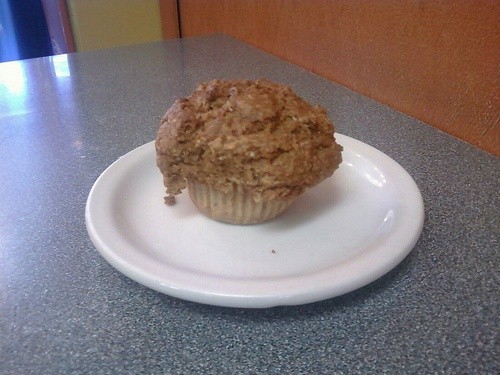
[84, 132, 425, 308]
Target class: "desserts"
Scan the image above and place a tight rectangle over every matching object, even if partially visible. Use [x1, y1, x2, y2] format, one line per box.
[154, 78, 343, 225]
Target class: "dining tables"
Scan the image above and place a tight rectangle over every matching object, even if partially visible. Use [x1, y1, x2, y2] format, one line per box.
[0, 32, 500, 374]
